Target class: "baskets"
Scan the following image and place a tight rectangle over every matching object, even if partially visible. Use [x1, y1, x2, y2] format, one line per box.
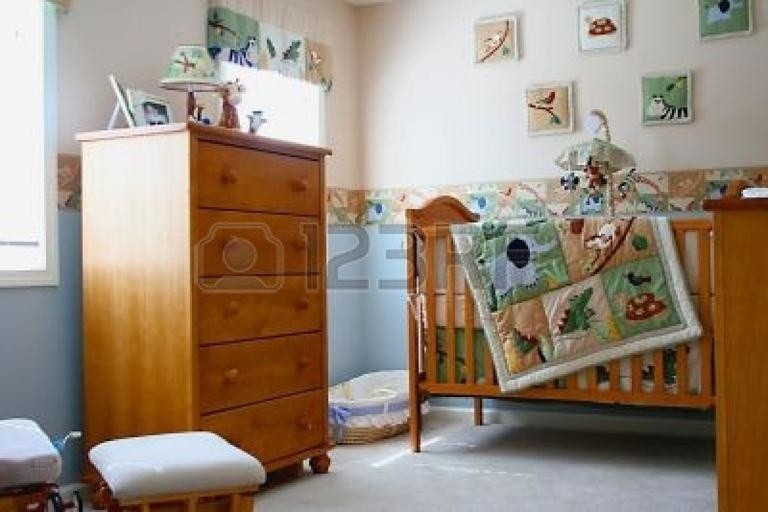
[327, 368, 432, 445]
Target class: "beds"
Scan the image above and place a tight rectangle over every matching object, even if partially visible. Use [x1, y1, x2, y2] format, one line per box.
[405, 195, 718, 453]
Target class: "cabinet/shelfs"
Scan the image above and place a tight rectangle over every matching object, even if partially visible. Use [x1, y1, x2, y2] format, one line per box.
[702, 195, 768, 512]
[76, 121, 332, 512]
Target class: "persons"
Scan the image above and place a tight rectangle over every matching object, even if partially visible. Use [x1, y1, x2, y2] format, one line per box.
[216, 80, 248, 130]
[143, 101, 167, 125]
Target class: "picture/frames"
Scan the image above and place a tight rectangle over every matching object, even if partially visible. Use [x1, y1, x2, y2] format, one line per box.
[640, 70, 693, 126]
[697, 0, 754, 42]
[474, 16, 520, 64]
[577, 0, 627, 56]
[107, 72, 174, 129]
[523, 80, 574, 135]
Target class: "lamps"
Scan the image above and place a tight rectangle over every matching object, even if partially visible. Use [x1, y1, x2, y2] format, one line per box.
[157, 43, 226, 125]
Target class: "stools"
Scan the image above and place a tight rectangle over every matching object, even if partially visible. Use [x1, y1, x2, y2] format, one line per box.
[0, 418, 66, 512]
[84, 429, 267, 512]
